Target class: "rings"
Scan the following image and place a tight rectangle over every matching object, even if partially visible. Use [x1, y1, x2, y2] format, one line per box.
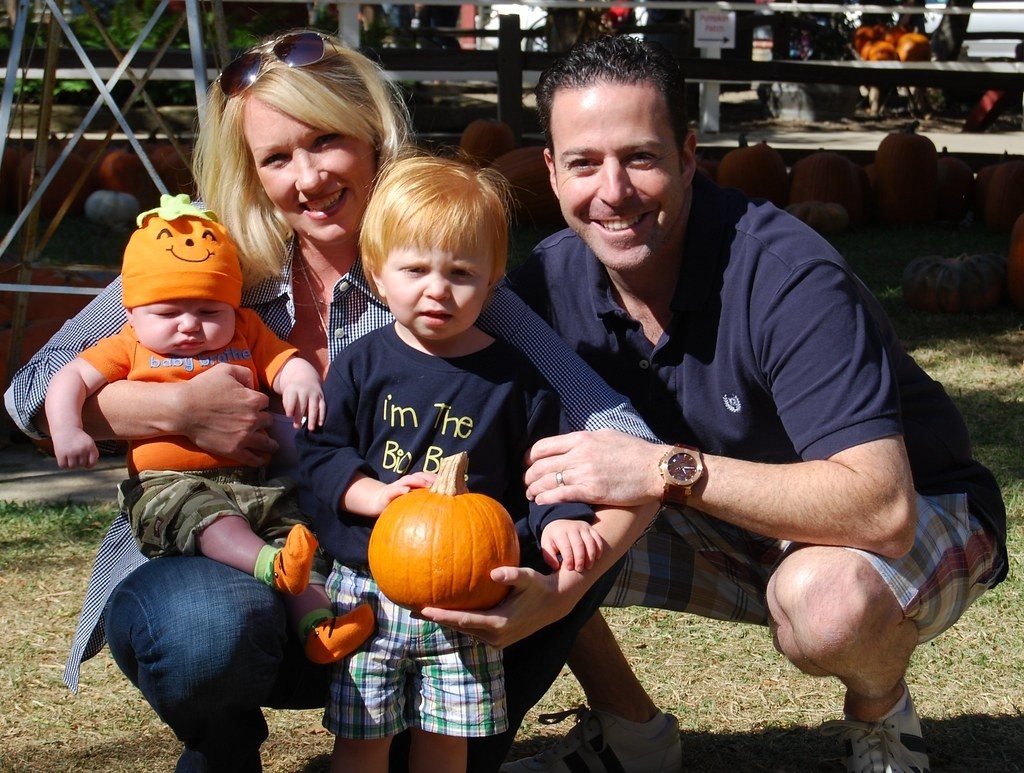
[553, 471, 567, 486]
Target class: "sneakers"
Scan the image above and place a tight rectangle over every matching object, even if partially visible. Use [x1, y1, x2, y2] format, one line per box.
[818, 675, 930, 773]
[500, 703, 682, 773]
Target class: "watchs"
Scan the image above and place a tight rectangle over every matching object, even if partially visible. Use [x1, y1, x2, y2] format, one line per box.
[657, 443, 706, 510]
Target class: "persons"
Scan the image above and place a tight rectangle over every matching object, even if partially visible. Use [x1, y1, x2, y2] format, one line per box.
[495, 31, 1008, 773]
[288, 154, 603, 772]
[5, 29, 663, 773]
[42, 195, 375, 663]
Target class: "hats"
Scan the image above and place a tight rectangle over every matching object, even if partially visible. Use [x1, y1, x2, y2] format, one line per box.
[122, 193, 242, 311]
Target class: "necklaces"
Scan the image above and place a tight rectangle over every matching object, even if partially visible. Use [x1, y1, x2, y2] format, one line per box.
[294, 244, 332, 338]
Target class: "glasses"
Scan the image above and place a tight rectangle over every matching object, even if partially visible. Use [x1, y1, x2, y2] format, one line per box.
[215, 32, 338, 98]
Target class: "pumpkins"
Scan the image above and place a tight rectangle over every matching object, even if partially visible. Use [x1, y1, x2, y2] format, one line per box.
[1, 15, 1024, 393]
[369, 452, 518, 609]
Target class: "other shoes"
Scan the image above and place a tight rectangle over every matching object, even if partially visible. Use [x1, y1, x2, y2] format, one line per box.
[174, 748, 262, 773]
